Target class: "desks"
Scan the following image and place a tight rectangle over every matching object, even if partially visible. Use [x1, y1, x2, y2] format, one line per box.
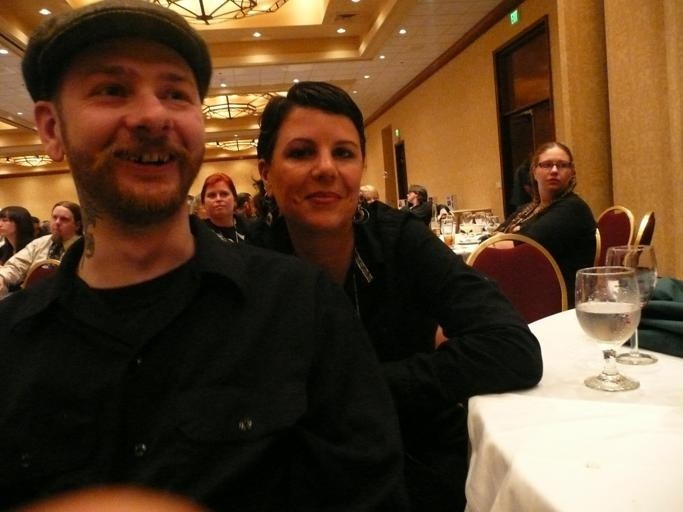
[463, 308, 683, 512]
[437, 231, 497, 264]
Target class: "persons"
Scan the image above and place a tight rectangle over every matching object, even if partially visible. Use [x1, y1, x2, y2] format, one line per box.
[0, 0, 411, 511]
[494, 143, 597, 310]
[248, 81, 543, 512]
[186, 174, 275, 245]
[360, 185, 433, 225]
[1, 200, 84, 295]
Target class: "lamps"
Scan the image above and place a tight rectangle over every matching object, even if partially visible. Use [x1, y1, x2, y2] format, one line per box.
[216, 139, 255, 152]
[6, 154, 53, 168]
[200, 91, 278, 119]
[150, 0, 288, 25]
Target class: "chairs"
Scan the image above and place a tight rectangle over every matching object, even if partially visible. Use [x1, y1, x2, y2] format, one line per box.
[20, 259, 59, 290]
[465, 205, 655, 324]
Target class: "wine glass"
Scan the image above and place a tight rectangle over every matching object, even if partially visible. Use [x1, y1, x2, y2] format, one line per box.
[461, 212, 473, 239]
[605, 245, 659, 364]
[475, 212, 485, 224]
[485, 212, 493, 225]
[489, 216, 500, 234]
[575, 265, 641, 391]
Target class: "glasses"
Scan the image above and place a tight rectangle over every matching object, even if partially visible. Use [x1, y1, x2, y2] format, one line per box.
[539, 160, 573, 168]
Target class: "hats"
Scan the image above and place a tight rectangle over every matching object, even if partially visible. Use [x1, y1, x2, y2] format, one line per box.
[22, 0, 211, 102]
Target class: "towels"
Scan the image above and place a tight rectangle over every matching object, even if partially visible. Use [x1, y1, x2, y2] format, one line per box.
[617, 272, 683, 358]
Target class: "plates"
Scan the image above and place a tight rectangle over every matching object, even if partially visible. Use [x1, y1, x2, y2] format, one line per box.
[458, 240, 481, 244]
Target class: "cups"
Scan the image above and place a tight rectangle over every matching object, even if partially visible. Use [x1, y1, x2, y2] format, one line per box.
[445, 215, 458, 233]
[442, 223, 456, 248]
[432, 222, 440, 237]
